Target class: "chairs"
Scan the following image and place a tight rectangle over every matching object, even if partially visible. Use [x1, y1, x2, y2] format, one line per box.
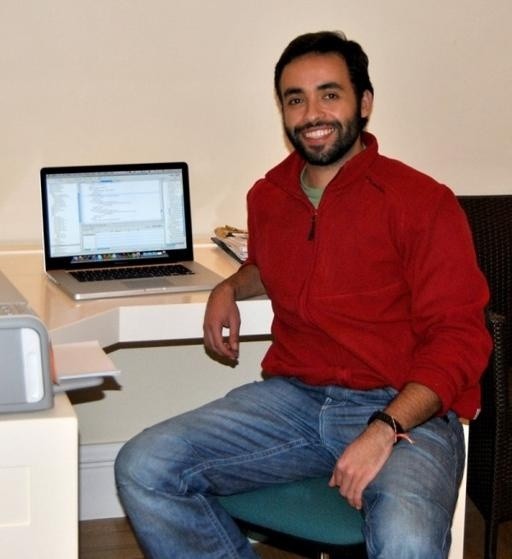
[216, 193, 512, 559]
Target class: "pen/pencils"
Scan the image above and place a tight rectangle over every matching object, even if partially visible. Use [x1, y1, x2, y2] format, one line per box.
[227, 232, 249, 238]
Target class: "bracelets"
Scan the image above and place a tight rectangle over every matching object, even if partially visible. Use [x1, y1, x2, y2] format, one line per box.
[367, 411, 404, 446]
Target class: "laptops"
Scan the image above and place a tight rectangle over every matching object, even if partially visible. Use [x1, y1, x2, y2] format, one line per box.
[40, 161, 226, 300]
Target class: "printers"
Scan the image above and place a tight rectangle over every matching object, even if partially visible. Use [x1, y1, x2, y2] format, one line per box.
[1, 266, 121, 414]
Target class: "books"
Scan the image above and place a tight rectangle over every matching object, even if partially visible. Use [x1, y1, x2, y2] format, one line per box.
[210, 227, 249, 266]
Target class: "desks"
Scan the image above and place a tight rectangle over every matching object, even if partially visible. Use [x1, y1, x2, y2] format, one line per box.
[0, 243, 278, 558]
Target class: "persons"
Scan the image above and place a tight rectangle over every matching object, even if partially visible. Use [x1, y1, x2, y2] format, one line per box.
[112, 29, 494, 559]
